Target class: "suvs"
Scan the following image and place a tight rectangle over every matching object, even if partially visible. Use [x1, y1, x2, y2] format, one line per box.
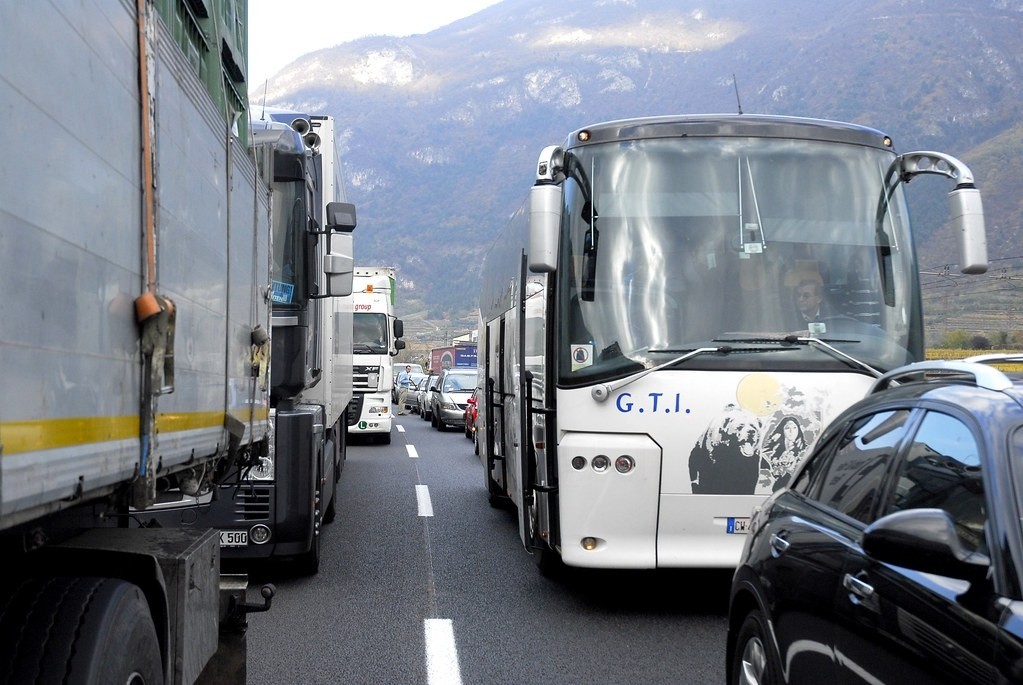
[429, 366, 480, 433]
[727, 350, 1023, 685]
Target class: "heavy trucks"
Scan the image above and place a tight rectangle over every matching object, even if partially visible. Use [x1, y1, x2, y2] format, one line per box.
[131, 100, 359, 586]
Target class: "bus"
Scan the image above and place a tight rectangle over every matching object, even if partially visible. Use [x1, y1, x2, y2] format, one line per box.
[469, 108, 992, 588]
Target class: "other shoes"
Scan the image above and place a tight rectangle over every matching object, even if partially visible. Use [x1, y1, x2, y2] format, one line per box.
[398, 412, 408, 415]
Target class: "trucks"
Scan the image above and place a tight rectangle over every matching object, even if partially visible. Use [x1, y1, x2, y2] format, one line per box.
[333, 264, 406, 443]
[0, 0, 278, 685]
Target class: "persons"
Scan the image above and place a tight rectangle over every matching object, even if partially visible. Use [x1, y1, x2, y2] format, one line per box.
[428, 369, 435, 378]
[787, 280, 838, 331]
[398, 365, 411, 416]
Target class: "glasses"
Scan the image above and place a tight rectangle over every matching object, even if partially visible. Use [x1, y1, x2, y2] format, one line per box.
[796, 292, 814, 299]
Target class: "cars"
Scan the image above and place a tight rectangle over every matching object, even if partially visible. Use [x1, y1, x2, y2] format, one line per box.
[392, 362, 439, 421]
[463, 386, 479, 456]
